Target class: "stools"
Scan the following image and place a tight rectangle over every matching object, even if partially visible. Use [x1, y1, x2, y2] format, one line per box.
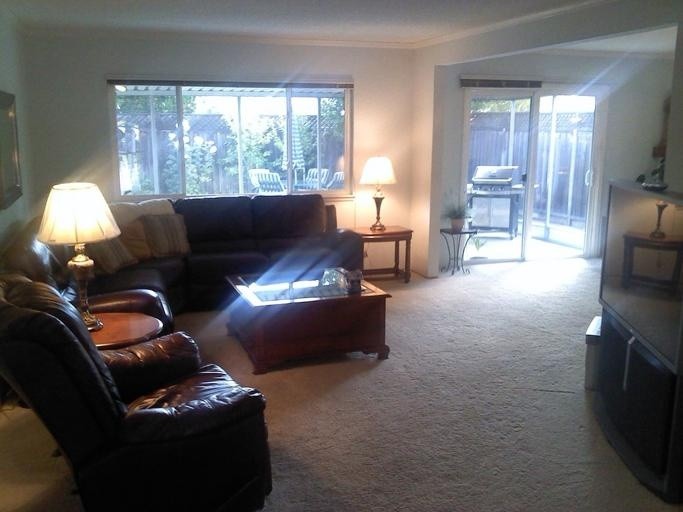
[440, 229, 477, 276]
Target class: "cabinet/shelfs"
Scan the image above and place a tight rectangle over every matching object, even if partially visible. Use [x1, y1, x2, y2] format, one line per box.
[585, 182, 683, 503]
[466, 188, 522, 240]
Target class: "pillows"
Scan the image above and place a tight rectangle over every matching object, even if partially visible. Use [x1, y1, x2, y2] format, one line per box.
[85, 198, 191, 275]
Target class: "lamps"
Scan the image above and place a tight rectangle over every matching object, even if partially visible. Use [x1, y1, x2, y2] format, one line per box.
[35, 182, 122, 330]
[359, 156, 398, 231]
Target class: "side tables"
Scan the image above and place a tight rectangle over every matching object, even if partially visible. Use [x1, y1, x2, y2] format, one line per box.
[80, 312, 163, 349]
[350, 226, 413, 282]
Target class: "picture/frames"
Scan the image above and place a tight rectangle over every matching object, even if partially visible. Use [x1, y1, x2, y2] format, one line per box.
[0, 90, 23, 209]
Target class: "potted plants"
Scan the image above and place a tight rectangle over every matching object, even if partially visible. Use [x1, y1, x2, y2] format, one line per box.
[441, 186, 477, 231]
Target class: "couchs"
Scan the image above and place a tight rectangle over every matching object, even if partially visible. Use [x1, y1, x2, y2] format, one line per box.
[0, 194, 363, 333]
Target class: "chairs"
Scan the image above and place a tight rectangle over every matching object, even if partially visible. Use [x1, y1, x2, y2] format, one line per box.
[247, 168, 344, 195]
[0, 272, 272, 512]
